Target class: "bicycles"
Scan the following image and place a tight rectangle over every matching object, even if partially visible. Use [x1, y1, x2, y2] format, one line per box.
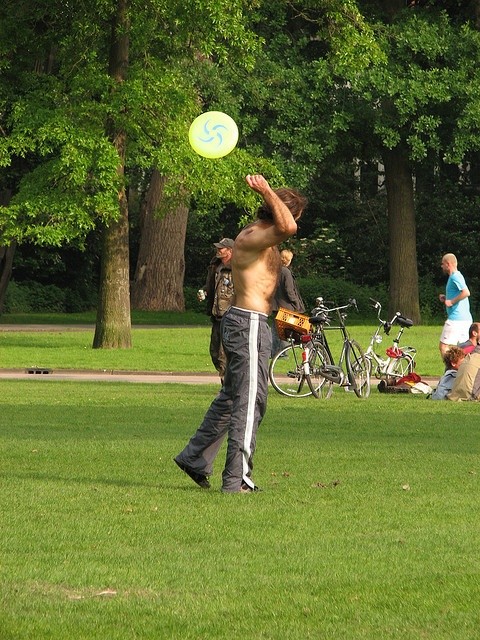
[302, 298, 371, 397]
[269, 297, 334, 398]
[344, 297, 417, 392]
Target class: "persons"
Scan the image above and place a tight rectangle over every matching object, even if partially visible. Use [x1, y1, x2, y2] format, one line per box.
[270, 249, 305, 357]
[430, 347, 465, 400]
[429, 322, 480, 401]
[196, 238, 234, 388]
[173, 174, 306, 494]
[438, 251, 473, 355]
[444, 348, 480, 402]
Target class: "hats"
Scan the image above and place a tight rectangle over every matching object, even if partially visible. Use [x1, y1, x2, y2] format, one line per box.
[213, 238, 234, 249]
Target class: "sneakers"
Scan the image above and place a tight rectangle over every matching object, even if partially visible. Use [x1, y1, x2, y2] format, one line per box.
[223, 480, 258, 492]
[173, 455, 211, 489]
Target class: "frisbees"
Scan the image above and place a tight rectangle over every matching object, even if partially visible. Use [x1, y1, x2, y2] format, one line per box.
[189, 111, 239, 158]
